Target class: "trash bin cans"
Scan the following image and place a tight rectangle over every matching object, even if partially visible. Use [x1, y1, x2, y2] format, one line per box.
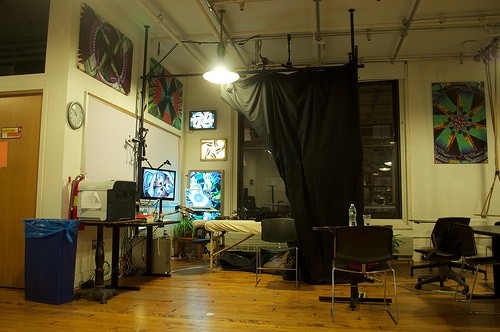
[21, 218, 78, 305]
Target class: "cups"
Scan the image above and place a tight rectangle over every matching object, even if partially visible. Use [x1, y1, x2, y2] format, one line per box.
[147, 216, 153, 224]
[363, 215, 371, 226]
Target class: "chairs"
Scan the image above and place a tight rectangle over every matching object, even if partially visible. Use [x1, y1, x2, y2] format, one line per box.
[256, 218, 302, 288]
[192, 228, 215, 259]
[410, 216, 489, 296]
[318, 227, 399, 325]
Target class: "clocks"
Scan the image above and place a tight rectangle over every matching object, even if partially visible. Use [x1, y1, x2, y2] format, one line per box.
[68, 101, 86, 129]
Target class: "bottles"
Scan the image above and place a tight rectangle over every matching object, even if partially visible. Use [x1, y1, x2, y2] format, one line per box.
[348, 204, 356, 227]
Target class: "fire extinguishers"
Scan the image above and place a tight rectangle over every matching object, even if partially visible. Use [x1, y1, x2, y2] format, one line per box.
[69, 170, 87, 230]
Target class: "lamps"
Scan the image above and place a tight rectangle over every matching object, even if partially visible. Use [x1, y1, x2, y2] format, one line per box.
[202, 10, 241, 84]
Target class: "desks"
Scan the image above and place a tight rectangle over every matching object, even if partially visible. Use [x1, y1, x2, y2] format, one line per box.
[407, 218, 435, 225]
[312, 225, 393, 309]
[205, 219, 416, 275]
[76, 219, 181, 304]
[466, 225, 500, 299]
[191, 218, 226, 259]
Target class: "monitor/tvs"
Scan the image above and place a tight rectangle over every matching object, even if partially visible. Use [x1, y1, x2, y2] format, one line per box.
[140, 167, 176, 201]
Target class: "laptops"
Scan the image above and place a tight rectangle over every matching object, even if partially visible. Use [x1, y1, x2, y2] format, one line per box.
[185, 189, 219, 212]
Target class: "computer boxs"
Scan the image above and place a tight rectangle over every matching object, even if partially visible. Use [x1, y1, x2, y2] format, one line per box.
[131, 234, 171, 275]
